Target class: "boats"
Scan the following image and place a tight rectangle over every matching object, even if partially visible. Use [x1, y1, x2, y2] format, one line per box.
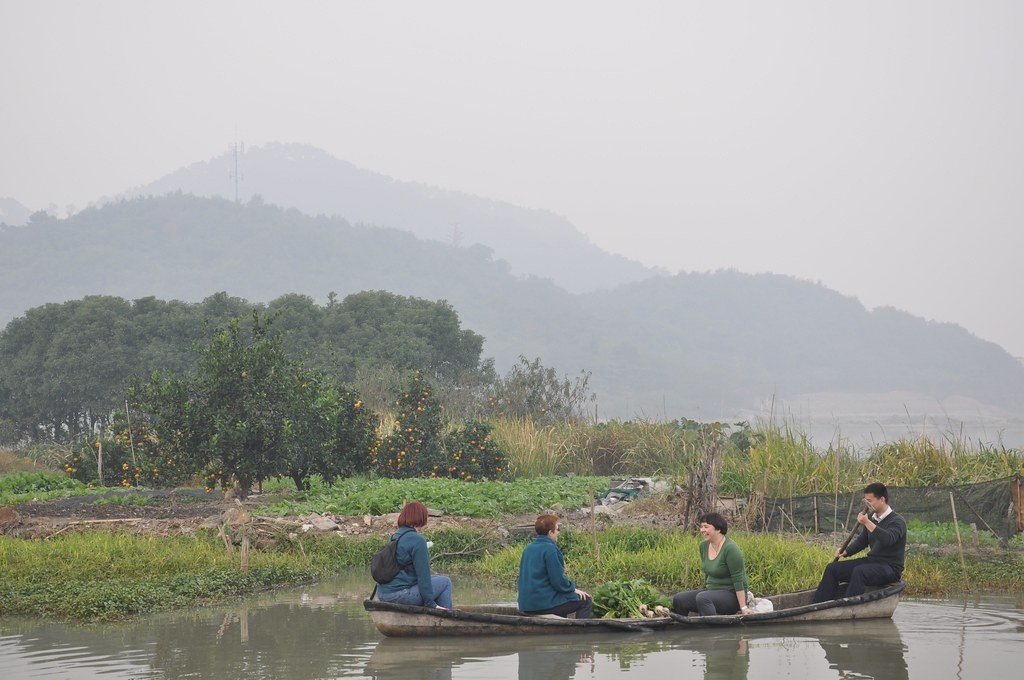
[365, 600, 674, 637]
[670, 581, 907, 625]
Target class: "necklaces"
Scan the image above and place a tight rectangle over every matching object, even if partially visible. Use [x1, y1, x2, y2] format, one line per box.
[713, 537, 725, 555]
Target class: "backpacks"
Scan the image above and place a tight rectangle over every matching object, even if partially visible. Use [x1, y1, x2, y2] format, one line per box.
[371, 529, 418, 584]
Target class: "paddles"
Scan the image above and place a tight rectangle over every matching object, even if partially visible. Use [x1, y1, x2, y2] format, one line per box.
[831, 497, 876, 563]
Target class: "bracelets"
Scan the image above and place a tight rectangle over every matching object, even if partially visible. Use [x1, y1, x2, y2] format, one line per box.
[741, 606, 747, 611]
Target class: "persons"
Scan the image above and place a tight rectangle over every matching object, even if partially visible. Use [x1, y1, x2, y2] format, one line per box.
[377, 503, 452, 610]
[518, 516, 592, 619]
[814, 482, 906, 603]
[672, 513, 753, 616]
